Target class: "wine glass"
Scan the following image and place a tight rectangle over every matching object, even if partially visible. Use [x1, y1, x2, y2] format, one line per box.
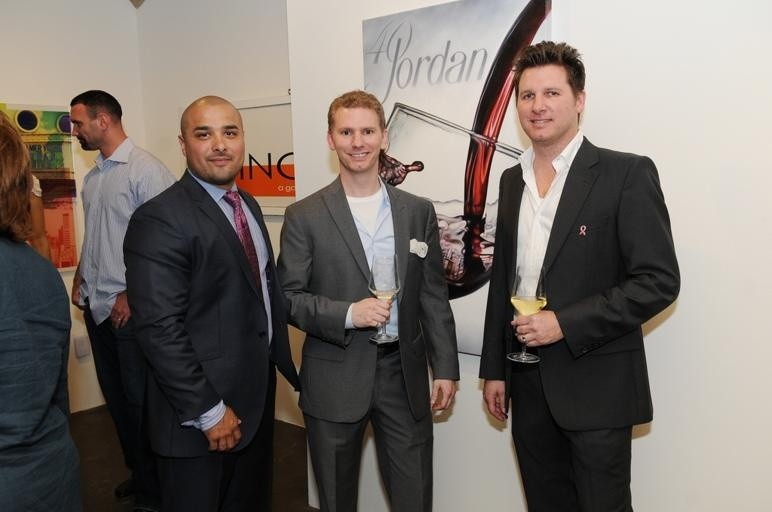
[368, 255, 400, 343]
[376, 103, 521, 299]
[507, 267, 547, 364]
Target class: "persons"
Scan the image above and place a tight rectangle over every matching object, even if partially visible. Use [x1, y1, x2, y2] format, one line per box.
[68, 90, 188, 503]
[121, 97, 298, 512]
[277, 89, 461, 511]
[481, 40, 682, 512]
[3, 110, 78, 509]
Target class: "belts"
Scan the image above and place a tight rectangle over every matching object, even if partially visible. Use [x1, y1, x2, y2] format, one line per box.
[377, 343, 400, 354]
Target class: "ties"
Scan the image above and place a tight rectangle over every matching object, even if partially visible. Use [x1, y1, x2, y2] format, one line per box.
[223, 192, 265, 299]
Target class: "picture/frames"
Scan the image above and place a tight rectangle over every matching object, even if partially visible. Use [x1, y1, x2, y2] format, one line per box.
[232, 94, 298, 217]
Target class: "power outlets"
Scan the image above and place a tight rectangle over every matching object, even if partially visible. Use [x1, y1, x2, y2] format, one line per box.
[73, 336, 92, 359]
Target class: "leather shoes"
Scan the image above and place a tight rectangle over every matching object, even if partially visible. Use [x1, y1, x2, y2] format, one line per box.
[114, 473, 139, 497]
